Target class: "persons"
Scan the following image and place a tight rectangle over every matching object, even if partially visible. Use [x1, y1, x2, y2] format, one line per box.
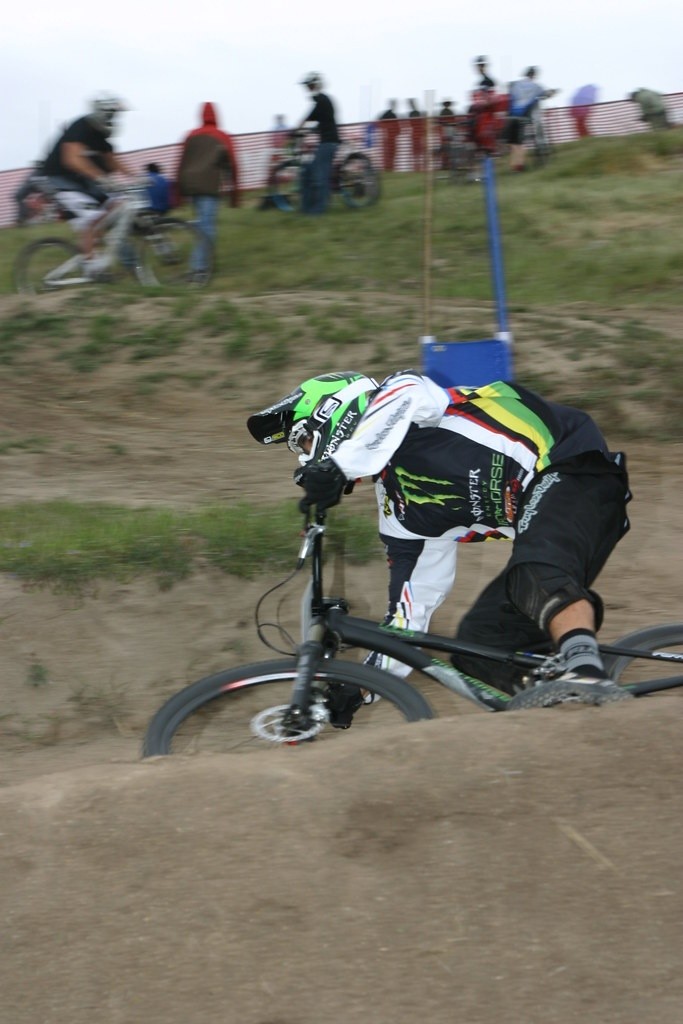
[177, 101, 239, 289]
[438, 99, 459, 169]
[409, 98, 425, 172]
[249, 368, 633, 711]
[141, 162, 170, 217]
[508, 66, 558, 171]
[294, 70, 343, 214]
[378, 99, 402, 171]
[43, 98, 152, 279]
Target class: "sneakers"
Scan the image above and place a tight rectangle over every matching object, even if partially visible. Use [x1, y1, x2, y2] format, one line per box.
[531, 664, 626, 702]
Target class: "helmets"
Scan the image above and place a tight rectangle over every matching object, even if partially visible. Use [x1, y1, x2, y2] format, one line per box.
[87, 95, 126, 136]
[247, 371, 379, 495]
[299, 70, 324, 97]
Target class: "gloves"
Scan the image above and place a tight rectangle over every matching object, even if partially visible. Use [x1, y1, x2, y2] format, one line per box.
[323, 683, 364, 731]
[293, 458, 347, 514]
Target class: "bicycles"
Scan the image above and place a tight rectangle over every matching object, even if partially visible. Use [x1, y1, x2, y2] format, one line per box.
[11, 174, 216, 296]
[268, 127, 382, 211]
[141, 505, 683, 762]
